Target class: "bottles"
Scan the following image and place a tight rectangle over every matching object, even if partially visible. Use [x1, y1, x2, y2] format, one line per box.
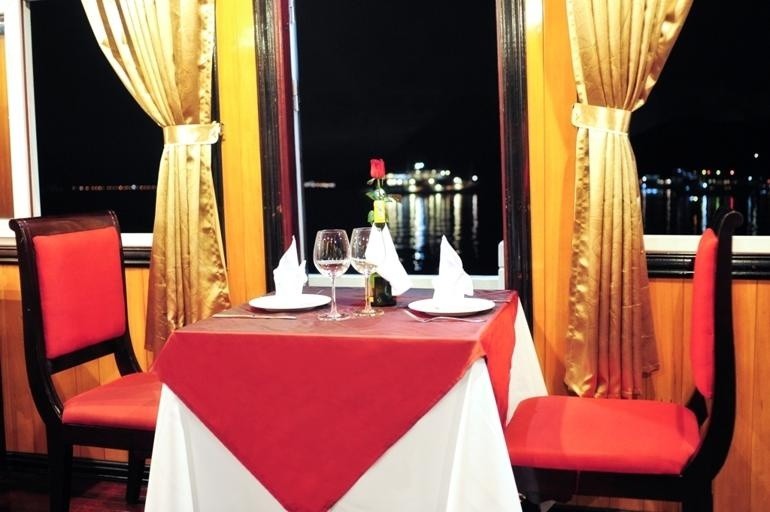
[367, 197, 397, 307]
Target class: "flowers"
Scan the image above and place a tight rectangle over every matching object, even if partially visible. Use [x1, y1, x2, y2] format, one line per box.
[366, 158, 394, 227]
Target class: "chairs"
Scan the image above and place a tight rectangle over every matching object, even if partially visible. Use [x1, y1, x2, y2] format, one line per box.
[497, 209, 743, 512]
[9, 211, 162, 511]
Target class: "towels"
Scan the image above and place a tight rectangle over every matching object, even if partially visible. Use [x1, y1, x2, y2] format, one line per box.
[273, 235, 308, 306]
[431, 234, 473, 310]
[364, 222, 413, 297]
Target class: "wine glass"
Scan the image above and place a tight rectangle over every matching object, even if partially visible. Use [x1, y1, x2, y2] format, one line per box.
[313, 227, 385, 320]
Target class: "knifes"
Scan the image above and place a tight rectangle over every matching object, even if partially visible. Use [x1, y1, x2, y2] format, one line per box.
[213, 314, 297, 321]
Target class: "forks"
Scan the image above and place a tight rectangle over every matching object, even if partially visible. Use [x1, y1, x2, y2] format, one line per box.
[402, 307, 486, 324]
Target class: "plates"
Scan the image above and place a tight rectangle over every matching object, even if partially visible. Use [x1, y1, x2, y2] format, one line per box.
[408, 297, 496, 318]
[248, 293, 331, 311]
